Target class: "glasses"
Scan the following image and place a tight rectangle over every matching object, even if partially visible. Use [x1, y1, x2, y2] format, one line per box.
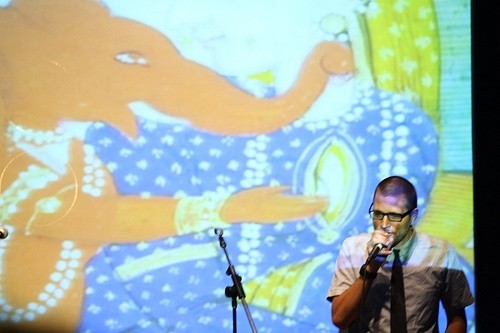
[368, 202, 414, 222]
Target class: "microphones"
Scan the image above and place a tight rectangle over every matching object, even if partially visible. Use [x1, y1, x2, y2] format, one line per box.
[0, 226, 8, 239]
[366, 243, 383, 265]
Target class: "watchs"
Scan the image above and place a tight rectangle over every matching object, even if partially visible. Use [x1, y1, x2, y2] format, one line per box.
[359, 264, 377, 280]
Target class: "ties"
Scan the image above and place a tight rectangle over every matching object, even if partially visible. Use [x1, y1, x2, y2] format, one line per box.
[390, 249, 407, 333]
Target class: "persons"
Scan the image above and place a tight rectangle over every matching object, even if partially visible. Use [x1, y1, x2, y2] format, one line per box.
[326, 176, 475, 333]
